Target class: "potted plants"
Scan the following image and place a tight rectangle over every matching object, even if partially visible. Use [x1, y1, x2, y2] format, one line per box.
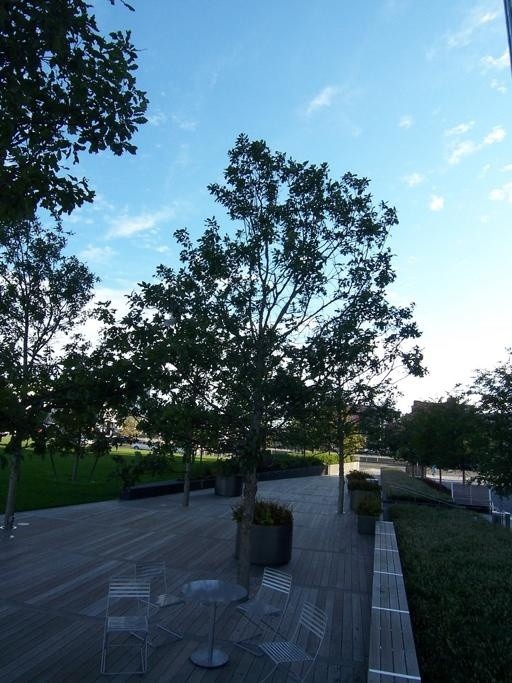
[355, 492, 382, 533]
[346, 469, 375, 495]
[207, 456, 245, 496]
[348, 478, 380, 511]
[233, 498, 294, 565]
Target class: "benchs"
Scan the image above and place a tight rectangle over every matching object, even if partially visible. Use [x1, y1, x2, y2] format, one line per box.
[120, 474, 210, 501]
[366, 518, 424, 682]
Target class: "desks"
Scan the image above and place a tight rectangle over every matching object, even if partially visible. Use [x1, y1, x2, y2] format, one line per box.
[181, 577, 250, 669]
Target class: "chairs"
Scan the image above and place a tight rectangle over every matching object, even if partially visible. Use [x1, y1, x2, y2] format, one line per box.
[228, 563, 298, 659]
[130, 560, 188, 647]
[98, 575, 151, 677]
[255, 600, 333, 681]
